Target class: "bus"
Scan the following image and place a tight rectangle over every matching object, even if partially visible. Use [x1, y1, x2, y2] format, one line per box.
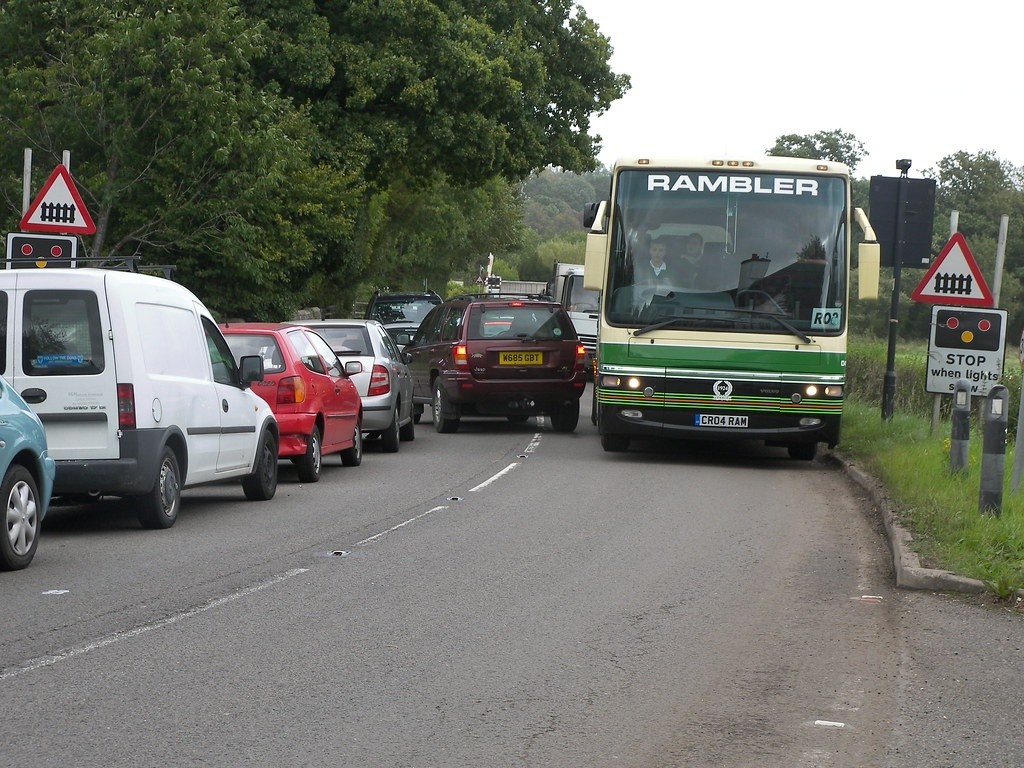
[585, 153, 879, 461]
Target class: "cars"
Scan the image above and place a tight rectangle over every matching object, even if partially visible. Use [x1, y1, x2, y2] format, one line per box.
[0, 373, 59, 572]
[214, 322, 363, 483]
[277, 316, 415, 453]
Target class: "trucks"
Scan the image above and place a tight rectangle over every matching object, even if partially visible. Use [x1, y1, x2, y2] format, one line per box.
[542, 258, 602, 381]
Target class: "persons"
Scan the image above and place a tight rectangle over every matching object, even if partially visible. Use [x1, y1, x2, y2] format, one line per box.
[631, 229, 832, 336]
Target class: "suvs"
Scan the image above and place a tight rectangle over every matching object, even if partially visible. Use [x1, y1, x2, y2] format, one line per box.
[0, 254, 279, 534]
[363, 288, 445, 354]
[399, 294, 587, 434]
